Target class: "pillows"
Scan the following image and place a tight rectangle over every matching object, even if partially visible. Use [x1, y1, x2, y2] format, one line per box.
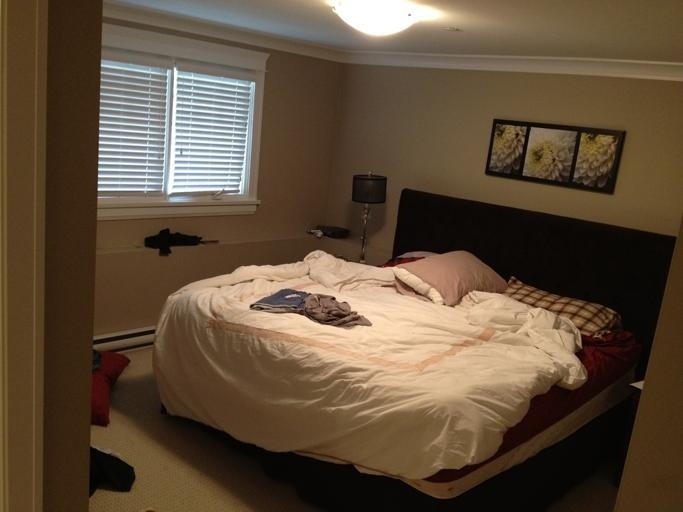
[499, 276, 621, 339]
[92, 352, 130, 427]
[392, 250, 508, 307]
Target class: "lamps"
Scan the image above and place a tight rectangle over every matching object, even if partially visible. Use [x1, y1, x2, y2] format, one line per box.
[352, 171, 387, 264]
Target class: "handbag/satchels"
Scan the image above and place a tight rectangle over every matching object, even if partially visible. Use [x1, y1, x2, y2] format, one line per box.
[317, 225, 351, 239]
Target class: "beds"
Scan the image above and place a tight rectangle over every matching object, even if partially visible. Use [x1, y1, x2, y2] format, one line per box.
[153, 188, 677, 512]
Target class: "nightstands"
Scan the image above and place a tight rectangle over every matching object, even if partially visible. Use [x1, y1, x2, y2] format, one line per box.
[616, 380, 643, 487]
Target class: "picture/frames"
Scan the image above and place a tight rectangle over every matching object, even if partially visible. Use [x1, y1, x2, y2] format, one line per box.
[486, 119, 625, 194]
